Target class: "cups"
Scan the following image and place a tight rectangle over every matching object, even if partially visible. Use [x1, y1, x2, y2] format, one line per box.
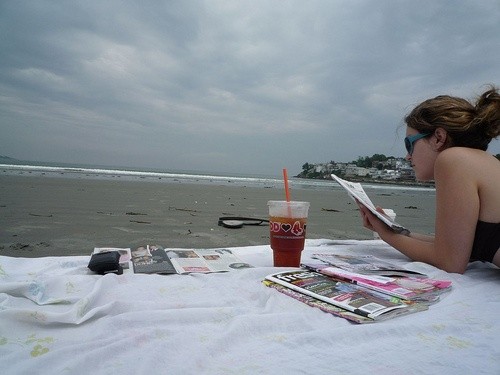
[267, 200, 310, 268]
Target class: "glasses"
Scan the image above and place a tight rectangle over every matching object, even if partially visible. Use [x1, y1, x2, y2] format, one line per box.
[404, 132, 433, 156]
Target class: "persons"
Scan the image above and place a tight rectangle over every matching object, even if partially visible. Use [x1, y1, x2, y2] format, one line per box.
[360, 83, 500, 275]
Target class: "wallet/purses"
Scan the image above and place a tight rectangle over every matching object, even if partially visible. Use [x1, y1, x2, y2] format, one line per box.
[88, 252, 124, 276]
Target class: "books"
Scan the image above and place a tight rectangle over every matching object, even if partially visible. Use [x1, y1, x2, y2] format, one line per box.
[262, 253, 453, 325]
[331, 174, 410, 236]
[90, 244, 256, 276]
